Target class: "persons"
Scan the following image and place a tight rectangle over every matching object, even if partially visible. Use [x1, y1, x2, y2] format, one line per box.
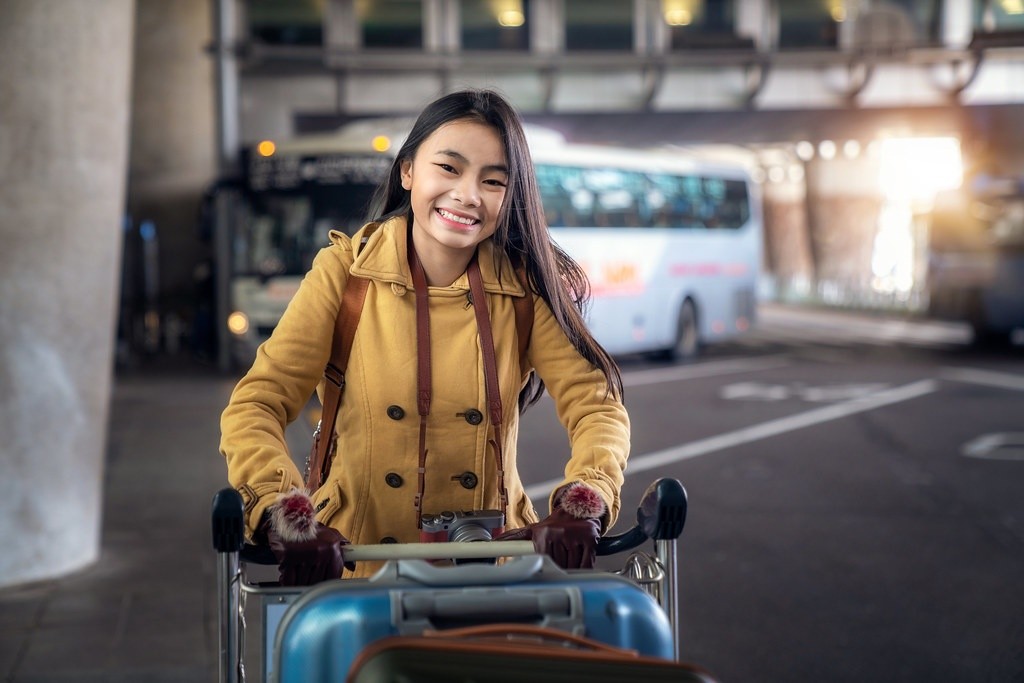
[219, 89, 631, 584]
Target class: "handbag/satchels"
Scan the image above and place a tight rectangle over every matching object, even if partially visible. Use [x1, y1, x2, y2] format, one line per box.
[345, 625, 716, 683]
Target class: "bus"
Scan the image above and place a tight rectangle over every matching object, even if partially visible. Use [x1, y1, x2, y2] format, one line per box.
[190, 118, 763, 368]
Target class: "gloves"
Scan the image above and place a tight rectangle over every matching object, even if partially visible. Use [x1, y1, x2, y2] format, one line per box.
[270, 492, 357, 588]
[493, 482, 605, 571]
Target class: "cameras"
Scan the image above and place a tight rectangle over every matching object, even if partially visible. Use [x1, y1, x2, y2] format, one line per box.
[423, 510, 507, 567]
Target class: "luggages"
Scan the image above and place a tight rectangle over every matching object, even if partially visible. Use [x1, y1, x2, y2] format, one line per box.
[271, 558, 672, 683]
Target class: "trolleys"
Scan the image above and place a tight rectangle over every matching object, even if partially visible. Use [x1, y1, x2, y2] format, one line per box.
[208, 475, 689, 683]
